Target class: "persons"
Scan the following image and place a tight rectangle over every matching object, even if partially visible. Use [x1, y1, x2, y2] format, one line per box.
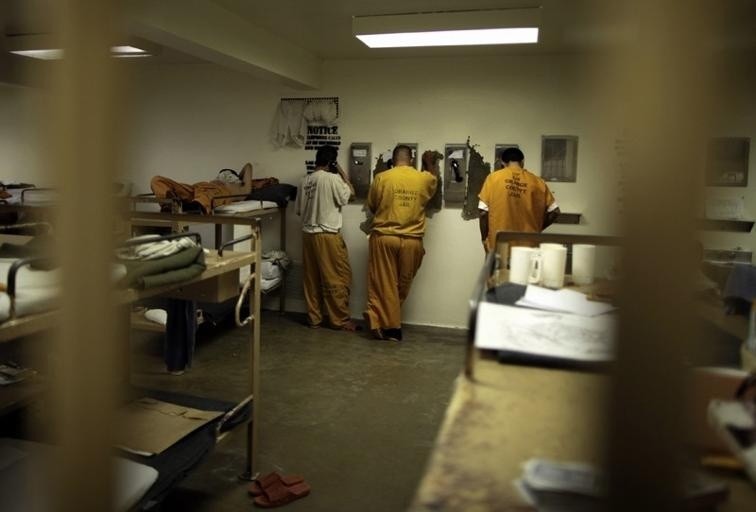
[151, 162, 253, 215]
[363, 143, 437, 341]
[478, 147, 559, 289]
[295, 145, 361, 332]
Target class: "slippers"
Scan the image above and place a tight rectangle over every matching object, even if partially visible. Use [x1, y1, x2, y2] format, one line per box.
[246, 469, 305, 495]
[332, 323, 363, 332]
[245, 479, 312, 508]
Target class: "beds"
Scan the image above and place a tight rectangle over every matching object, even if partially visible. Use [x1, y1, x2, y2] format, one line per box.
[122, 179, 287, 323]
[1, 184, 61, 214]
[405, 228, 755, 510]
[0, 223, 261, 512]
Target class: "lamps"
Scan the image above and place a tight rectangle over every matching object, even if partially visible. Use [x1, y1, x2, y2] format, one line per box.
[351, 8, 542, 52]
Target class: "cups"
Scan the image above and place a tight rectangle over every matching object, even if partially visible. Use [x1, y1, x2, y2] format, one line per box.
[571, 244, 596, 286]
[538, 242, 567, 288]
[509, 246, 542, 286]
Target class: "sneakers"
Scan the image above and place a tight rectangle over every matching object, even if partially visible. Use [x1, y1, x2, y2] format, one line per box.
[367, 327, 402, 342]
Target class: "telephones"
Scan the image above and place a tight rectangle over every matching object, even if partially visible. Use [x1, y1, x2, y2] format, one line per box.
[449, 158, 462, 184]
[329, 157, 338, 174]
[387, 158, 392, 170]
